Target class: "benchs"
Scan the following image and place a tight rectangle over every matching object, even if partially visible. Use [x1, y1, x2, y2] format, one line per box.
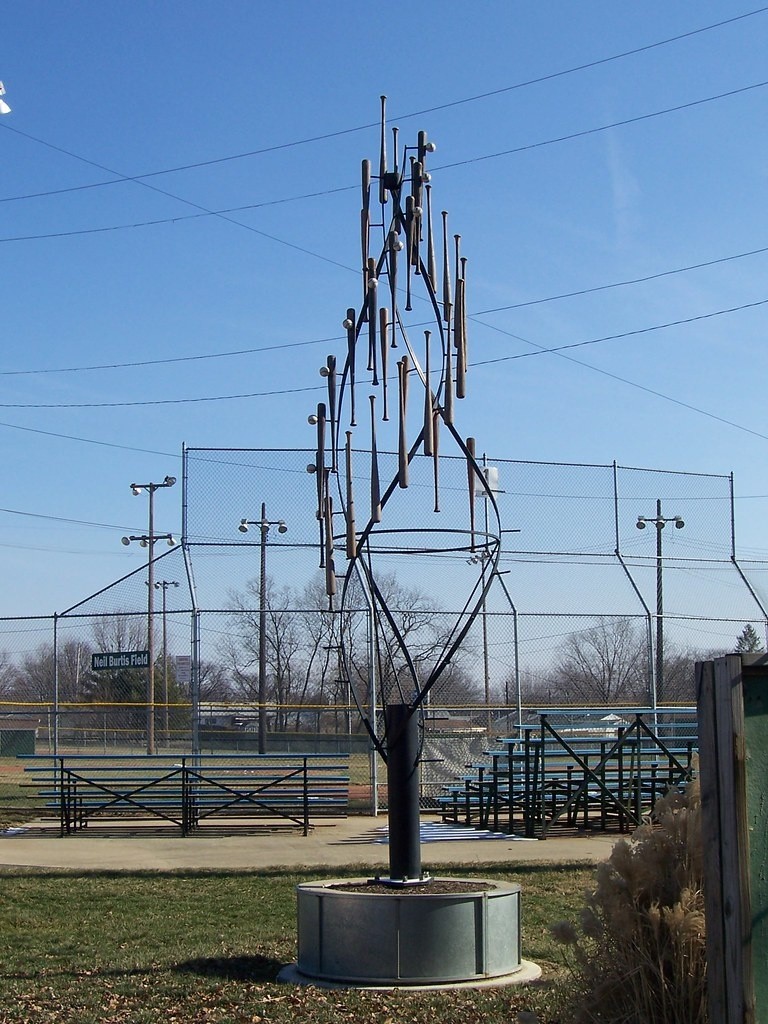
[17, 754, 350, 835]
[432, 707, 698, 841]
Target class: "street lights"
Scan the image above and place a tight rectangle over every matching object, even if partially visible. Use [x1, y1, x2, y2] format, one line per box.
[120, 476, 177, 760]
[636, 498, 684, 758]
[237, 501, 288, 755]
[144, 580, 180, 746]
